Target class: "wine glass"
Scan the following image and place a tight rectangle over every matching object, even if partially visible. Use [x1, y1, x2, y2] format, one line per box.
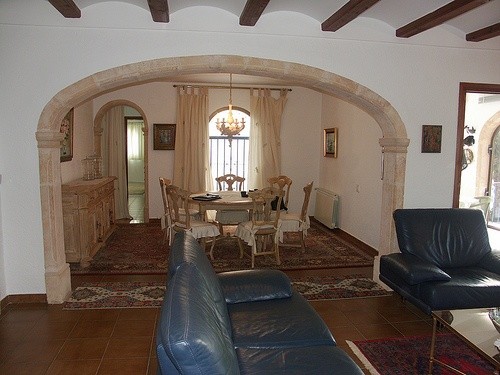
[488, 311, 500, 351]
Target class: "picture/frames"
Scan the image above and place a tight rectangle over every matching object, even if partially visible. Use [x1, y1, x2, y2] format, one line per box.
[60, 108, 74, 162]
[153, 123, 175, 150]
[323, 127, 337, 158]
[421, 125, 442, 154]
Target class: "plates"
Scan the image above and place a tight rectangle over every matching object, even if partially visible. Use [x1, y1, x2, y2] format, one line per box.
[192, 196, 216, 200]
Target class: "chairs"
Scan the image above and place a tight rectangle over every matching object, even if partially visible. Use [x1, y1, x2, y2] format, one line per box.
[470, 196, 492, 216]
[158, 176, 221, 260]
[216, 174, 246, 234]
[235, 176, 314, 268]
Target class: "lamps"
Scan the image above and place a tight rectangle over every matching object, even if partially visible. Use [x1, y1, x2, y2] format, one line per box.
[215, 73, 246, 146]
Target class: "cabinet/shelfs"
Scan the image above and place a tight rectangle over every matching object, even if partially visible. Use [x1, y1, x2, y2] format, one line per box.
[62, 176, 118, 268]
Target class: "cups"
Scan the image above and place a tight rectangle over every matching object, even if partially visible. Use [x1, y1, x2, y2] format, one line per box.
[86, 155, 103, 178]
[81, 159, 95, 180]
[241, 189, 258, 197]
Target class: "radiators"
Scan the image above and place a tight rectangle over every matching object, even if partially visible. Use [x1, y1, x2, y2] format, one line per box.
[314, 187, 339, 229]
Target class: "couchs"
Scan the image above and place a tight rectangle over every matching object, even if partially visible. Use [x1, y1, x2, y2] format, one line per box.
[156, 230, 364, 375]
[378, 207, 500, 332]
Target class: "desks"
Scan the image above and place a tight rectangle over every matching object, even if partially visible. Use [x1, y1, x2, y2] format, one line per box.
[188, 190, 276, 246]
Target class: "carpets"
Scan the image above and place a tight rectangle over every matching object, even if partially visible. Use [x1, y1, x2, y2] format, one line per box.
[62, 273, 392, 311]
[345, 334, 500, 375]
[70, 221, 374, 274]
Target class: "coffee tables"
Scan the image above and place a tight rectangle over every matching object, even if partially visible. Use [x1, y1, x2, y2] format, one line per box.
[426, 308, 500, 375]
